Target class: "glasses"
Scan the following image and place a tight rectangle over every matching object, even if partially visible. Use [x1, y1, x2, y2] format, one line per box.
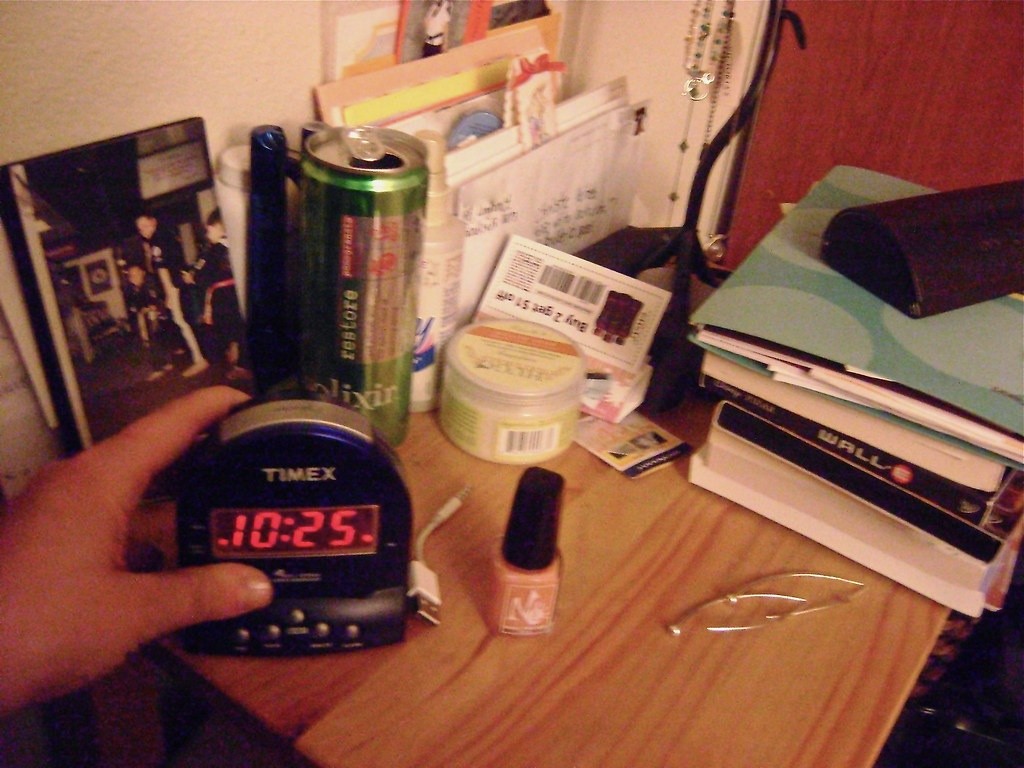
[668, 571, 869, 636]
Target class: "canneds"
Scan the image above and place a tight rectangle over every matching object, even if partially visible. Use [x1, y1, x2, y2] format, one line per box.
[295, 126, 429, 448]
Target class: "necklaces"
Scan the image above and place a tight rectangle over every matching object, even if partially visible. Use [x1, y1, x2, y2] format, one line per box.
[668, 0, 740, 226]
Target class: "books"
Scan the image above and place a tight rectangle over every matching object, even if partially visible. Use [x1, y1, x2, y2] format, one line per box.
[684, 163, 1024, 618]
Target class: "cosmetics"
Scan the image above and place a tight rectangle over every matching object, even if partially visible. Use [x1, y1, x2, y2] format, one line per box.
[484, 463, 567, 640]
[408, 127, 465, 416]
[436, 314, 587, 463]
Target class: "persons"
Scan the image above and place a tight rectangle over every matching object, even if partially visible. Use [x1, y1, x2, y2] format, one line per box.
[119, 206, 247, 384]
[421, 0, 452, 60]
[0, 384, 277, 721]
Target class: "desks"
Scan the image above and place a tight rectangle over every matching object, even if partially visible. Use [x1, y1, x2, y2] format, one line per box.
[85, 254, 951, 768]
